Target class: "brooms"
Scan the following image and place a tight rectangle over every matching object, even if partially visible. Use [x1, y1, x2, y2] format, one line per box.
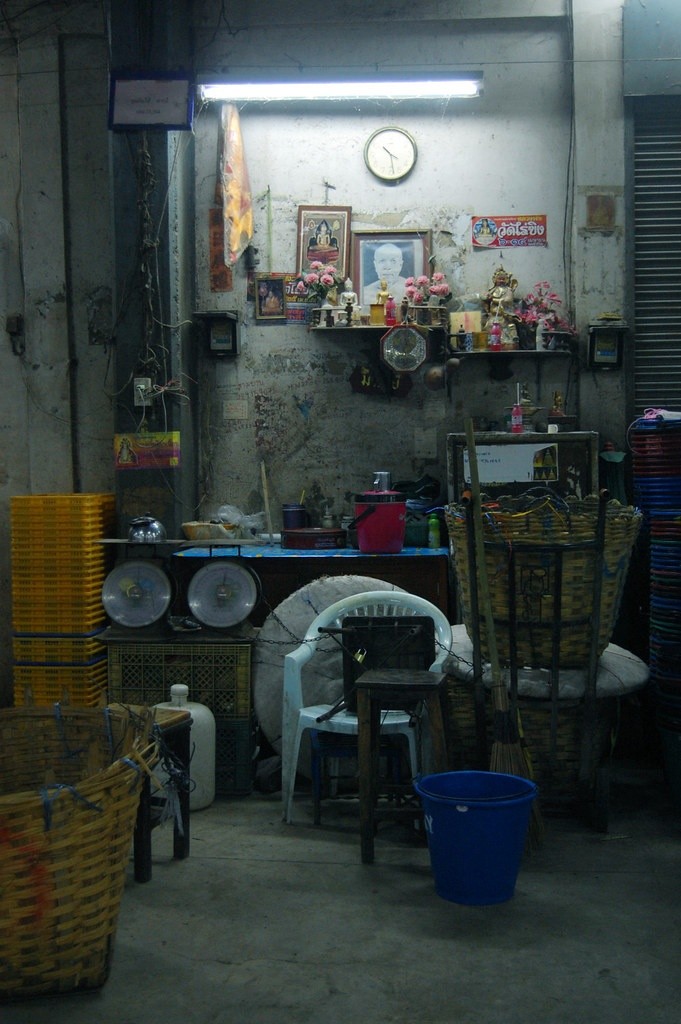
[463, 417, 546, 865]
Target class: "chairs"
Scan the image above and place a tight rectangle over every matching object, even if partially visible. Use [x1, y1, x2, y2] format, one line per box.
[466, 491, 622, 824]
[279, 589, 454, 833]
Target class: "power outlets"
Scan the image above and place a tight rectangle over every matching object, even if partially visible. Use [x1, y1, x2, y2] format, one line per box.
[133, 377, 154, 407]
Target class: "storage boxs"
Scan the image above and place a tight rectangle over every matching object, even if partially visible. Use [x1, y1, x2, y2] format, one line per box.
[113, 430, 182, 470]
[8, 491, 253, 796]
[281, 526, 347, 549]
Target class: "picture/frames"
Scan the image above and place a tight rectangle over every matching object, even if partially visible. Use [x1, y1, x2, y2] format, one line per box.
[254, 276, 287, 319]
[589, 330, 624, 370]
[350, 229, 434, 322]
[205, 318, 240, 357]
[297, 204, 353, 282]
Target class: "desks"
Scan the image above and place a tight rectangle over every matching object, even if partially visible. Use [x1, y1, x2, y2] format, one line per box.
[172, 543, 449, 617]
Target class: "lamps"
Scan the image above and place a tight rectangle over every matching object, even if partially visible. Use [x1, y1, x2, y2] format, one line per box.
[194, 69, 483, 100]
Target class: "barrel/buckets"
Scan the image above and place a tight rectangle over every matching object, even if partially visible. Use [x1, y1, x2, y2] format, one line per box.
[627, 407, 681, 803]
[348, 489, 406, 553]
[414, 770, 539, 904]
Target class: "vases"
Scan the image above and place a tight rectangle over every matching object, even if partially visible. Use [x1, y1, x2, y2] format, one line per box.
[319, 297, 336, 328]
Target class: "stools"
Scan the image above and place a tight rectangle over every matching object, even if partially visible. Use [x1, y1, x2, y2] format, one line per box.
[108, 701, 194, 883]
[311, 614, 451, 866]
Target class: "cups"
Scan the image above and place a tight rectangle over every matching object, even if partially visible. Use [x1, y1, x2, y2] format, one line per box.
[281, 503, 307, 529]
[458, 333, 473, 352]
[373, 471, 391, 492]
[473, 332, 488, 351]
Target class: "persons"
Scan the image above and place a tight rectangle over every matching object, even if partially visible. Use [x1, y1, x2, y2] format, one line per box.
[552, 390, 563, 412]
[263, 291, 281, 312]
[520, 384, 531, 403]
[339, 278, 361, 311]
[364, 243, 407, 304]
[377, 281, 391, 307]
[485, 270, 519, 341]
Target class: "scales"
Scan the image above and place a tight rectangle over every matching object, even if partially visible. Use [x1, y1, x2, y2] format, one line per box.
[180, 539, 264, 638]
[98, 540, 185, 637]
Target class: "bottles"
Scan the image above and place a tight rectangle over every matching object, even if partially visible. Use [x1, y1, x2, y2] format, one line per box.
[369, 303, 385, 325]
[154, 683, 216, 810]
[384, 296, 397, 326]
[323, 515, 355, 544]
[536, 319, 546, 350]
[490, 322, 501, 351]
[511, 404, 523, 433]
[428, 512, 440, 548]
[400, 297, 410, 324]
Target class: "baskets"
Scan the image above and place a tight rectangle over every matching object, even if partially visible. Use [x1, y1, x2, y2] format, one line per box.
[10, 493, 252, 799]
[443, 487, 644, 670]
[1, 703, 161, 1005]
[446, 675, 620, 815]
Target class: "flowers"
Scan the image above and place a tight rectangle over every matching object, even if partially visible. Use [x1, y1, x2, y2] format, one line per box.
[364, 126, 418, 184]
[297, 260, 345, 300]
[404, 271, 453, 307]
[513, 281, 577, 343]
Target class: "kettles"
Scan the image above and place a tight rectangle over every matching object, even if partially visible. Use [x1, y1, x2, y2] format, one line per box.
[128, 511, 166, 542]
